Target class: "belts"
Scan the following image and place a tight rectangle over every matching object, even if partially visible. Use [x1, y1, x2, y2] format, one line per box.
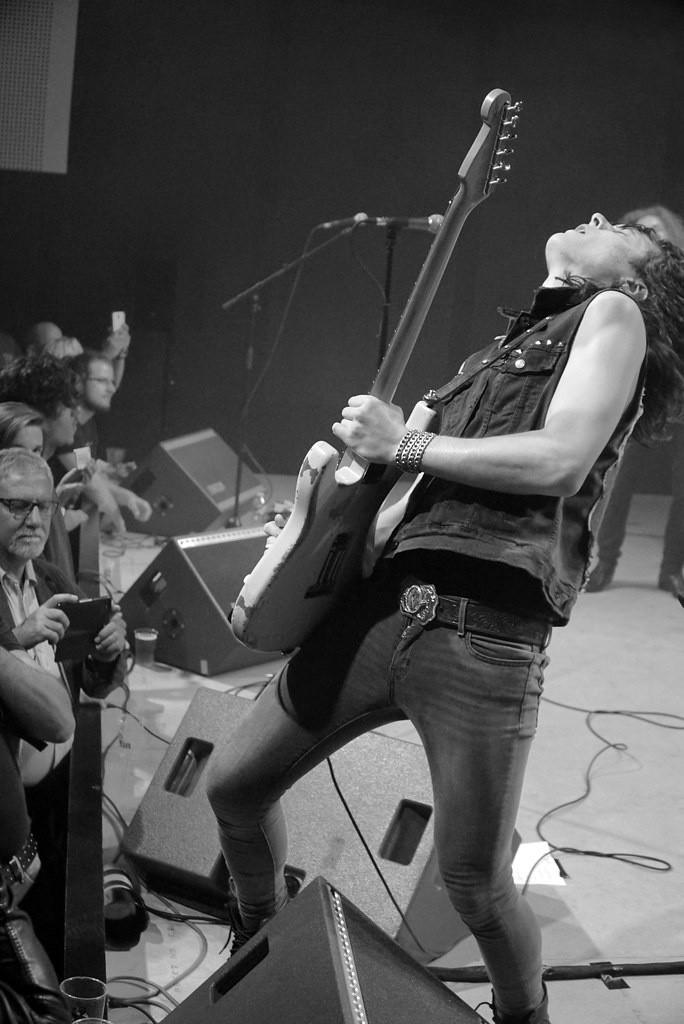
[0, 833, 38, 892]
[385, 571, 546, 646]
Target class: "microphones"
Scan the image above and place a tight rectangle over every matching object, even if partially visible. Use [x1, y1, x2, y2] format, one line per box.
[318, 212, 367, 229]
[364, 214, 444, 233]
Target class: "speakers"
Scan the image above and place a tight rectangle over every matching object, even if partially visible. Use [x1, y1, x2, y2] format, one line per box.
[117, 687, 490, 1024]
[116, 427, 300, 677]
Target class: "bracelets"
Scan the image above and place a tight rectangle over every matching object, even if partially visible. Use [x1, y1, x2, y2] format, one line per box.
[394, 430, 437, 473]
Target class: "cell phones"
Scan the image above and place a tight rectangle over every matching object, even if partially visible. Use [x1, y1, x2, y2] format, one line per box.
[55, 596, 112, 662]
[112, 311, 126, 333]
[73, 447, 90, 469]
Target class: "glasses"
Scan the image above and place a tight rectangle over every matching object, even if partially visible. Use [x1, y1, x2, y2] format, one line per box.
[91, 378, 116, 388]
[614, 223, 661, 247]
[0, 498, 59, 517]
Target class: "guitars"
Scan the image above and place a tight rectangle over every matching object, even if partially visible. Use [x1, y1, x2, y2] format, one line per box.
[231, 90, 523, 655]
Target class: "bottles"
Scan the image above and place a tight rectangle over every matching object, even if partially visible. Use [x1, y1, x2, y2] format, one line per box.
[251, 492, 265, 512]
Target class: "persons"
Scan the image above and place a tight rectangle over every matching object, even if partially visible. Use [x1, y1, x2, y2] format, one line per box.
[0, 322, 152, 1024]
[585, 206, 684, 599]
[206, 206, 684, 1024]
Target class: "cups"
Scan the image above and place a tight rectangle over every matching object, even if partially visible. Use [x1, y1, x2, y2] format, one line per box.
[59, 976, 108, 1024]
[71, 1017, 113, 1024]
[133, 626, 158, 668]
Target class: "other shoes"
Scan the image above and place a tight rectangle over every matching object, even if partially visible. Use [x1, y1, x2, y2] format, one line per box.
[474, 979, 549, 1024]
[220, 900, 269, 957]
